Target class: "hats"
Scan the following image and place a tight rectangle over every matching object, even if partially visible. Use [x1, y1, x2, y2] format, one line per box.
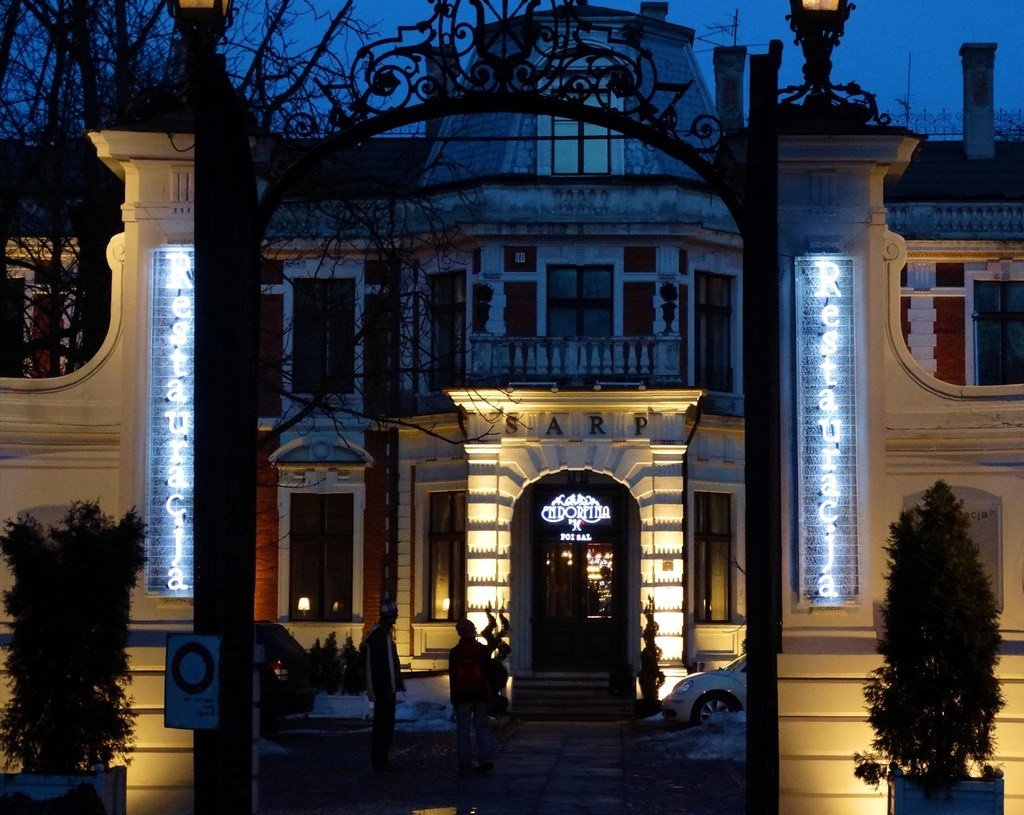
[456, 619, 476, 638]
[380, 597, 398, 620]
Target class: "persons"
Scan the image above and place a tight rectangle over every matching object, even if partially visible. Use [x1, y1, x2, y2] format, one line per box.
[449, 620, 495, 775]
[363, 600, 406, 774]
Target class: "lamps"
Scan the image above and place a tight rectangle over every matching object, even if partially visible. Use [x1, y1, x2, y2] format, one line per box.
[166, 0, 231, 96]
[776, 0, 876, 111]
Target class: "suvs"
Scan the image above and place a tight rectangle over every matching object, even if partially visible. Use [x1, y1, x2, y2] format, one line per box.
[255, 618, 316, 723]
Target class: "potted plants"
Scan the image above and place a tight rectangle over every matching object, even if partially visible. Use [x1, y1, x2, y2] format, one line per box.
[1, 506, 144, 803]
[478, 608, 511, 715]
[472, 282, 493, 333]
[660, 281, 678, 334]
[853, 482, 1003, 813]
[633, 600, 662, 719]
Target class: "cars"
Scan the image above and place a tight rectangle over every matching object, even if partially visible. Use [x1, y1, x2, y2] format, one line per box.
[659, 652, 747, 735]
